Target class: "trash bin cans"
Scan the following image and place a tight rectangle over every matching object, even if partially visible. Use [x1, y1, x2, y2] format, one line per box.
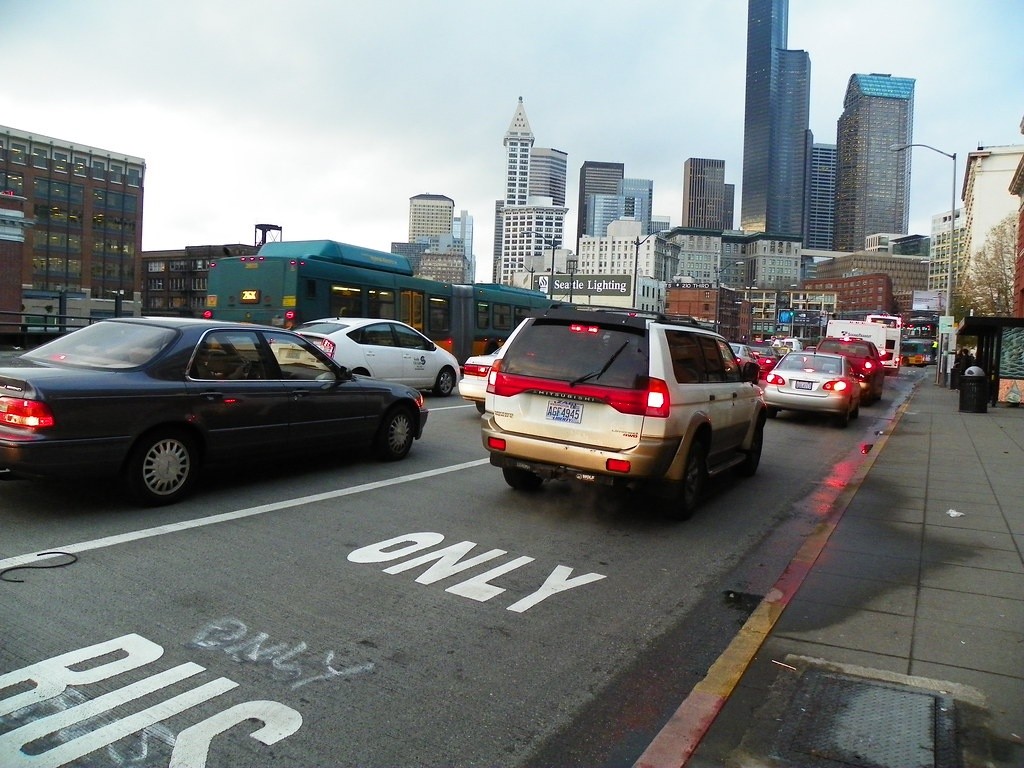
[958, 366, 988, 414]
[950, 363, 961, 389]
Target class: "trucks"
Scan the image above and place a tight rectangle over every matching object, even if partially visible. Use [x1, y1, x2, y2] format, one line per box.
[771, 337, 803, 357]
[825, 320, 887, 366]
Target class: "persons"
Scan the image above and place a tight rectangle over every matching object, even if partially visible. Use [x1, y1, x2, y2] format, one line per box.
[275, 317, 293, 330]
[836, 344, 841, 349]
[929, 339, 938, 360]
[954, 349, 974, 368]
[889, 320, 896, 328]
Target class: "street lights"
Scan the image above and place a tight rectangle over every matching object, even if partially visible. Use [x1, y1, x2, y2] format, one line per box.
[717, 262, 744, 332]
[521, 231, 555, 299]
[891, 144, 957, 388]
[632, 230, 660, 308]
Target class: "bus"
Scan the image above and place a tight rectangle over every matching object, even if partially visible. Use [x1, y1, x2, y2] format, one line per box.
[865, 313, 902, 375]
[204, 239, 577, 368]
[901, 338, 934, 367]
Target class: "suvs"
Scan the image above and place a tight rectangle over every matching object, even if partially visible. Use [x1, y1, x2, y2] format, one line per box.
[815, 335, 884, 404]
[480, 308, 767, 521]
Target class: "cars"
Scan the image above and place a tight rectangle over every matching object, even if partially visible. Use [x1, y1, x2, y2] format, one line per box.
[763, 350, 861, 426]
[270, 317, 461, 396]
[720, 343, 760, 384]
[751, 346, 781, 378]
[805, 346, 816, 353]
[458, 343, 503, 413]
[0, 317, 430, 508]
